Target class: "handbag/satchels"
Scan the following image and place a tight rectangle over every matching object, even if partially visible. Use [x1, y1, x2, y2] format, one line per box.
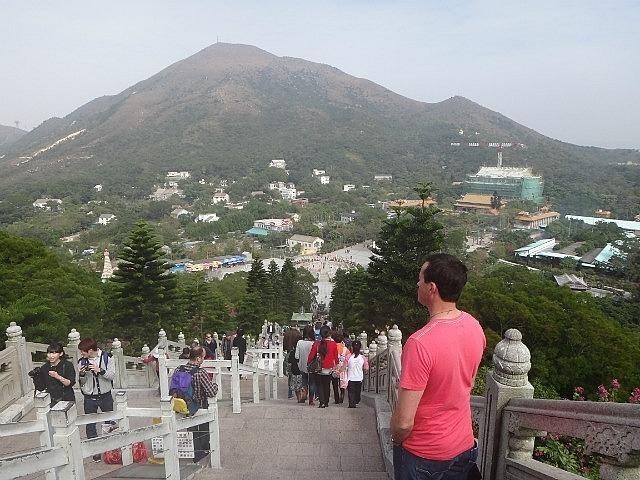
[307, 352, 322, 374]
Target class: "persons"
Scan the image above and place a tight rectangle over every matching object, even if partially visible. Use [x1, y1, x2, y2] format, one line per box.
[75, 337, 116, 463]
[390, 253, 486, 480]
[168, 347, 218, 462]
[140, 328, 246, 382]
[27, 343, 76, 406]
[260, 317, 370, 408]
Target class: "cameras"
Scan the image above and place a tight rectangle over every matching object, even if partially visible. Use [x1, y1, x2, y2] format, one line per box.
[28, 367, 41, 378]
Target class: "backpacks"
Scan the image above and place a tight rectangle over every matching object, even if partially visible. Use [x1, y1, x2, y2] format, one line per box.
[168, 365, 200, 417]
[282, 349, 293, 377]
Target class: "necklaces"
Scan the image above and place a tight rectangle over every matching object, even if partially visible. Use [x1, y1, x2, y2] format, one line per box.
[430, 309, 457, 319]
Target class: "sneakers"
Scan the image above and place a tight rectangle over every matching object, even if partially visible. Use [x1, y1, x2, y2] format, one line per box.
[287, 395, 359, 409]
[92, 454, 102, 463]
